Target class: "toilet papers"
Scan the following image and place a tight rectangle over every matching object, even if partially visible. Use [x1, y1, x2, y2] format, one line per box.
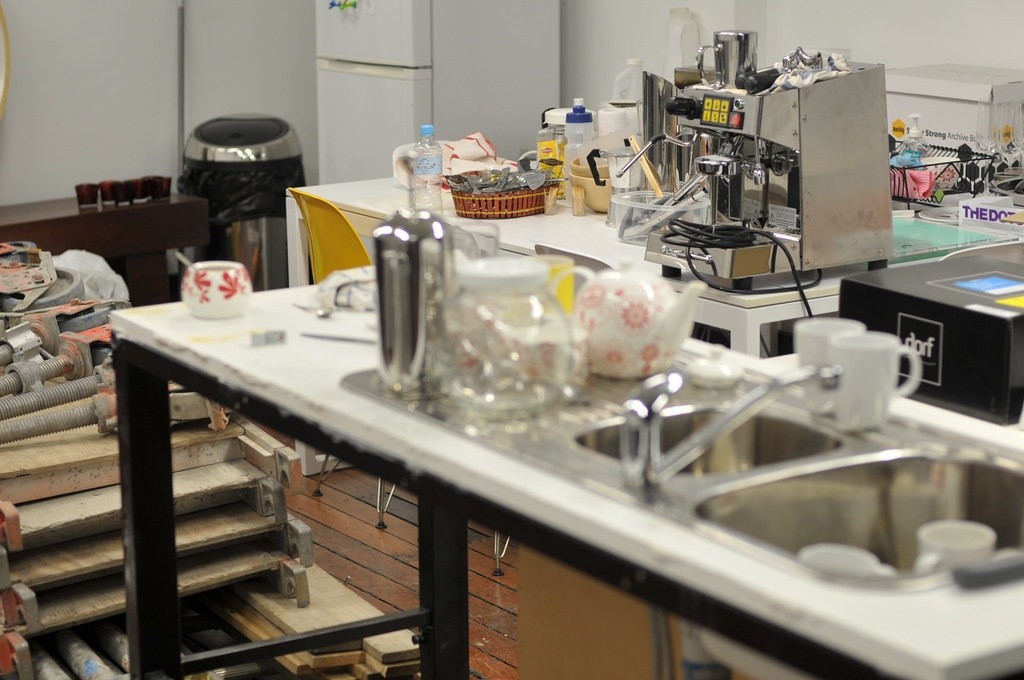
[597, 107, 640, 138]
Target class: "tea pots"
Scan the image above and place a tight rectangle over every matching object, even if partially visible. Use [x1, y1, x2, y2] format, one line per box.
[556, 258, 706, 379]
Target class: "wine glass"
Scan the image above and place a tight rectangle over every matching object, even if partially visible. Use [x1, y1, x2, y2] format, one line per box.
[976, 97, 1024, 197]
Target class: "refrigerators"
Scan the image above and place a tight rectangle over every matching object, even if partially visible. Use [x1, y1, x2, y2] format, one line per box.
[317, 0, 560, 265]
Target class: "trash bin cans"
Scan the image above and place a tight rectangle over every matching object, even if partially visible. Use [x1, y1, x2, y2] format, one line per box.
[177, 113, 307, 293]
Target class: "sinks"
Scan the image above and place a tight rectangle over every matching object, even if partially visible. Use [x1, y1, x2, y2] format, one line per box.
[676, 433, 1024, 597]
[508, 378, 864, 478]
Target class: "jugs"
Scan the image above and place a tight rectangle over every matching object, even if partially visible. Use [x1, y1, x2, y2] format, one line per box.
[587, 138, 647, 227]
[544, 108, 596, 176]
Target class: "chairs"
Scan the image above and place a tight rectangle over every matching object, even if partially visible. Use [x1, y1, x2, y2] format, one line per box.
[291, 188, 372, 287]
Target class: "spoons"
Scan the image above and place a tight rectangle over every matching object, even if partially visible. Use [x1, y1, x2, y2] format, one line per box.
[292, 302, 333, 317]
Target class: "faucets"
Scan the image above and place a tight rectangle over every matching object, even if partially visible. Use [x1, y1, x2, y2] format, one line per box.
[618, 360, 845, 505]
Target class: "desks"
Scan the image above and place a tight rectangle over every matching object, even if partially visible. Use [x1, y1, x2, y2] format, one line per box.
[1, 190, 211, 308]
[283, 177, 1024, 362]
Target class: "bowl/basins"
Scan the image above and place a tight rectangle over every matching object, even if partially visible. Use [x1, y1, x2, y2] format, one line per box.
[921, 157, 960, 191]
[611, 192, 710, 247]
[569, 158, 612, 212]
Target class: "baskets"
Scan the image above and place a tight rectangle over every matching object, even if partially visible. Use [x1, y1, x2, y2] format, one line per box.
[451, 187, 549, 218]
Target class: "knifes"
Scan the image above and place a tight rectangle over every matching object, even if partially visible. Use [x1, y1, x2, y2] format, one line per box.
[450, 157, 552, 193]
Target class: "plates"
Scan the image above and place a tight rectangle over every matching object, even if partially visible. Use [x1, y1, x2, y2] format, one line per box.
[918, 207, 960, 225]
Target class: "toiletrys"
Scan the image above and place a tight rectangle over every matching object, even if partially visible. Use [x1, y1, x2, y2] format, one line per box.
[899, 113, 933, 159]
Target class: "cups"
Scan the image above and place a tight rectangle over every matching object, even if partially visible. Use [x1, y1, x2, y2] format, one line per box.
[1004, 167, 1024, 206]
[799, 543, 896, 583]
[100, 175, 171, 206]
[75, 183, 100, 208]
[537, 255, 573, 313]
[913, 520, 997, 575]
[994, 172, 1021, 205]
[795, 316, 864, 415]
[831, 332, 923, 431]
[448, 222, 502, 257]
[181, 261, 250, 317]
[697, 30, 757, 89]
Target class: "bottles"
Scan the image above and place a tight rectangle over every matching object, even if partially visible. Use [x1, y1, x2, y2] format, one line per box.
[614, 59, 646, 135]
[412, 124, 441, 213]
[436, 259, 579, 413]
[564, 98, 594, 204]
